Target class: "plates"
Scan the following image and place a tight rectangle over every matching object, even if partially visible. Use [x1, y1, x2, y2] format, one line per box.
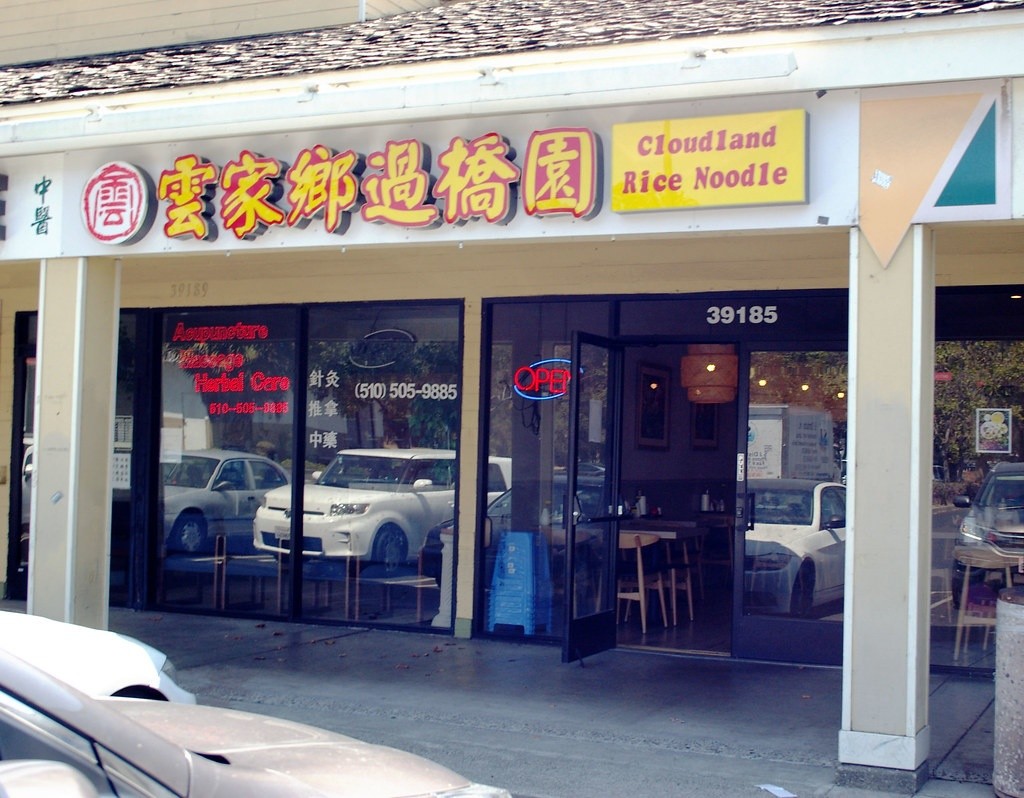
[981, 412, 1008, 439]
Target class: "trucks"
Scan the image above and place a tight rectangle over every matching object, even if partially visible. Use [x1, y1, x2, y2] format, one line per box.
[747, 403, 843, 510]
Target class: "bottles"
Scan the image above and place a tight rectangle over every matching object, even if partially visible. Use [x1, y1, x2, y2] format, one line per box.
[719, 500, 725, 511]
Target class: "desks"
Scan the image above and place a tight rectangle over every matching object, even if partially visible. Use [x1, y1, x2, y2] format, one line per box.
[576, 522, 706, 624]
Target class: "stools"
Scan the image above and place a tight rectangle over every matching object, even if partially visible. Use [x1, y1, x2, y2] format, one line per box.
[949, 544, 1024, 662]
[485, 531, 554, 639]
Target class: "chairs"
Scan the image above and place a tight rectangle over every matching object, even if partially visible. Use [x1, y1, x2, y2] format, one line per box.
[541, 515, 738, 635]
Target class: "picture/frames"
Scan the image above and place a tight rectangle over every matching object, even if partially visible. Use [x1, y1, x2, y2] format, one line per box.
[635, 362, 672, 450]
[689, 403, 720, 449]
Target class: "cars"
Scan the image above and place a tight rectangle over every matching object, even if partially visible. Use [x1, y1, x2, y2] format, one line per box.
[23, 435, 34, 524]
[0, 608, 510, 798]
[161, 447, 292, 554]
[951, 456, 1024, 605]
[742, 479, 846, 618]
[253, 449, 630, 589]
